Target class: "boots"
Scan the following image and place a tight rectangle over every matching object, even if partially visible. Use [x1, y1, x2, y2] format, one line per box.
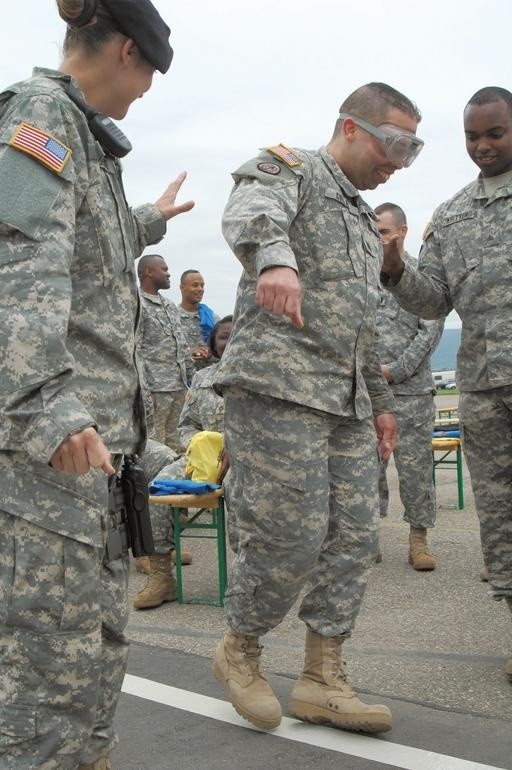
[133, 560, 177, 609]
[212, 632, 283, 729]
[170, 550, 193, 564]
[408, 525, 437, 570]
[135, 555, 160, 574]
[288, 630, 394, 733]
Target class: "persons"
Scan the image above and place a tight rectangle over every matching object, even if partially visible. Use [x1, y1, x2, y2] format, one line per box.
[175, 271, 220, 381]
[365, 202, 444, 570]
[134, 254, 186, 460]
[2, 1, 196, 769]
[133, 438, 192, 574]
[379, 85, 511, 687]
[211, 81, 423, 733]
[133, 315, 240, 609]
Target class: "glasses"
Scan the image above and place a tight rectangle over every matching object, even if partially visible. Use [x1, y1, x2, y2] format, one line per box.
[338, 111, 425, 170]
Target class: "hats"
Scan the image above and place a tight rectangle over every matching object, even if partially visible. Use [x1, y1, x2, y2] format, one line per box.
[102, 0, 175, 74]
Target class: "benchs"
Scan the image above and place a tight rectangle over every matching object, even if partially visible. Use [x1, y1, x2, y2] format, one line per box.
[436, 407, 460, 425]
[434, 419, 460, 433]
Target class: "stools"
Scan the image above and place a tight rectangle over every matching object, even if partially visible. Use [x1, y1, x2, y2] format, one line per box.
[149, 486, 226, 607]
[431, 438, 464, 510]
